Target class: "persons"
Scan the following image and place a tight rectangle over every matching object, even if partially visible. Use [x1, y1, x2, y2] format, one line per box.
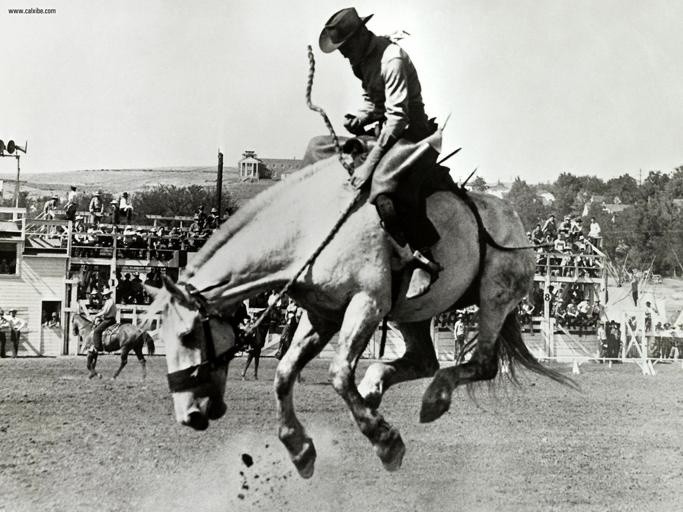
[297, 6, 441, 301]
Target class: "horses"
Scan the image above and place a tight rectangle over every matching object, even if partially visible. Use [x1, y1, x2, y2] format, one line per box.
[134, 152, 582, 480]
[71, 313, 155, 381]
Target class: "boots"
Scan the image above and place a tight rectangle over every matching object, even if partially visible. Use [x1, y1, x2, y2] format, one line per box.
[405, 249, 439, 301]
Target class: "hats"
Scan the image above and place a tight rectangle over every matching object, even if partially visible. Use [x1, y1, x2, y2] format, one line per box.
[319, 7, 374, 53]
[101, 289, 113, 295]
